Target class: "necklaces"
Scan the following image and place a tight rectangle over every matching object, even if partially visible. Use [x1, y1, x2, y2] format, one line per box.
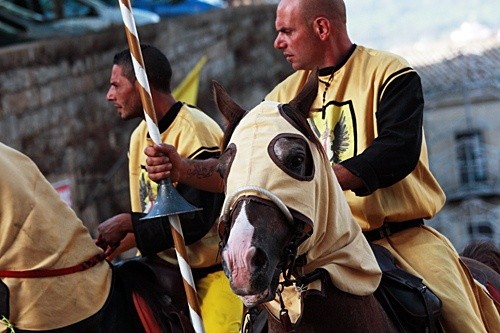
[318, 45, 337, 119]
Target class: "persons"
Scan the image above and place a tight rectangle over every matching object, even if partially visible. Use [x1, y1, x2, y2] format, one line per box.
[144, 0, 499, 333]
[94, 44, 241, 333]
[0, 142, 119, 333]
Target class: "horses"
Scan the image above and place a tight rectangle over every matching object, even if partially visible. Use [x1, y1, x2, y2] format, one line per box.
[0, 141, 223, 333]
[212, 66, 500, 333]
